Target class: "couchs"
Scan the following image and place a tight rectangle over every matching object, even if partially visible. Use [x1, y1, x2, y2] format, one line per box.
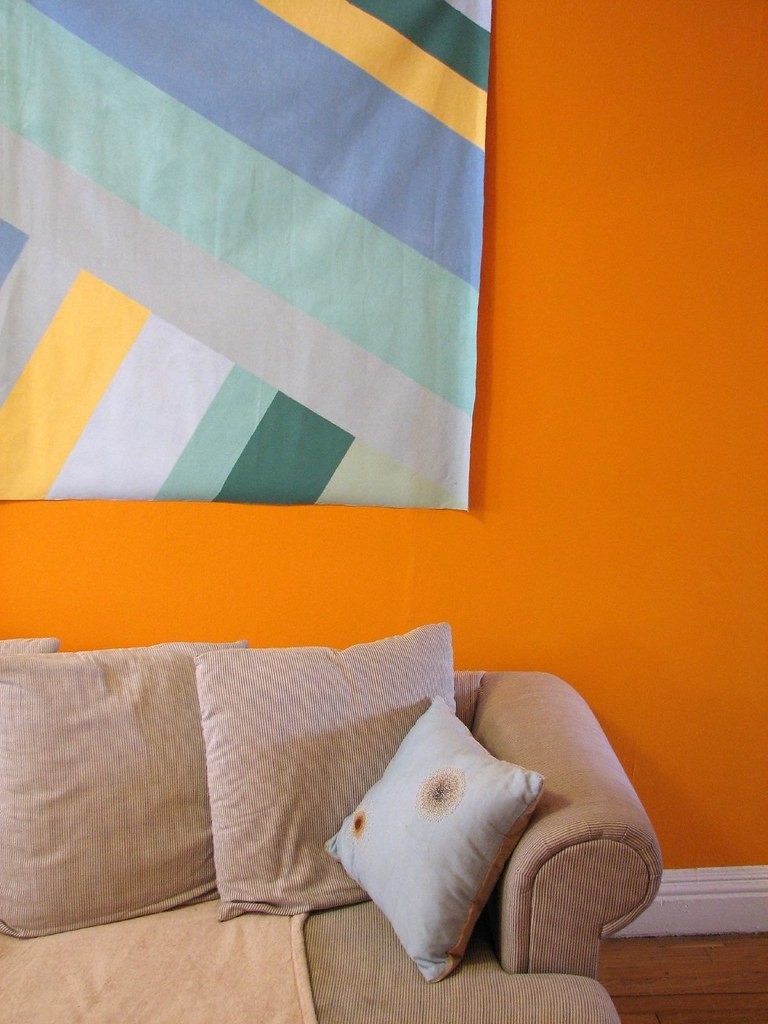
[0, 671, 663, 1024]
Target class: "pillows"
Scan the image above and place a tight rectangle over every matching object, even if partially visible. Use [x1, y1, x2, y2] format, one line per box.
[192, 617, 458, 920]
[0, 637, 61, 654]
[0, 640, 252, 941]
[327, 694, 547, 980]
